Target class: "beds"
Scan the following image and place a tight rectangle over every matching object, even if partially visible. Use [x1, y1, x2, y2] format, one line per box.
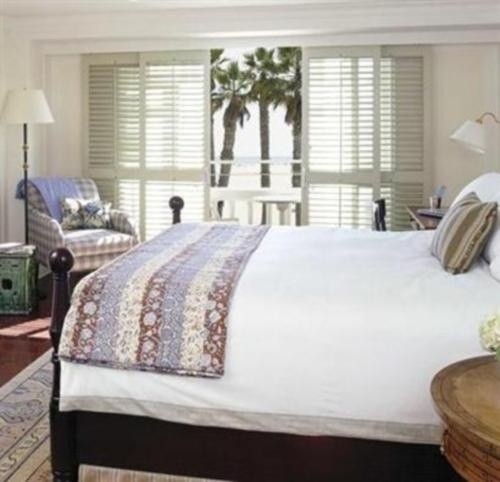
[50, 172, 500, 481]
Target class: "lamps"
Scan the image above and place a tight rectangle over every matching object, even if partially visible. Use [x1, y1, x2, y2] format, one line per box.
[0, 88, 55, 245]
[448, 111, 500, 154]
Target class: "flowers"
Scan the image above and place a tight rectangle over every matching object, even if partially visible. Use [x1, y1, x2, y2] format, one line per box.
[480, 316, 500, 350]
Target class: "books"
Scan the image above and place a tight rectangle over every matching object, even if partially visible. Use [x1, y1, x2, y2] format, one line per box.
[0, 243, 37, 255]
[417, 207, 448, 217]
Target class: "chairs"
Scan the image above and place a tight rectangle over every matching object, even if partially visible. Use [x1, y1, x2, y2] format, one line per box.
[373, 198, 387, 232]
[18, 178, 137, 295]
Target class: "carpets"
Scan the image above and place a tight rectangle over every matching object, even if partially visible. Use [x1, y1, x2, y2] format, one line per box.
[0, 346, 197, 482]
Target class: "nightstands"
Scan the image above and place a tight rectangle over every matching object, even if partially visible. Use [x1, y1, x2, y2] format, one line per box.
[407, 206, 454, 229]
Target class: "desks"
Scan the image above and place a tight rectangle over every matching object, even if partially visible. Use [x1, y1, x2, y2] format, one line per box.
[429, 355, 500, 482]
[0, 243, 38, 315]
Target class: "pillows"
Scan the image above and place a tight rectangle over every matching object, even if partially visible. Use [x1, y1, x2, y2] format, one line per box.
[58, 198, 114, 230]
[429, 192, 498, 273]
